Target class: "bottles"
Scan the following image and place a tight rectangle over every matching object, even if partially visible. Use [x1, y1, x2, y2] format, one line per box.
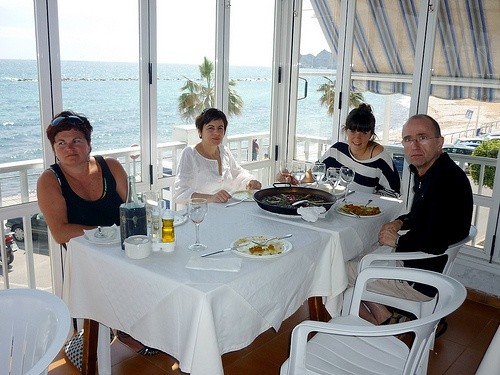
[151, 206, 175, 243]
[123, 175, 141, 208]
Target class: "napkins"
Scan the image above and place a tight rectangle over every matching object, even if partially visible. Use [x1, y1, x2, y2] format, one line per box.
[186, 256, 241, 272]
[297, 206, 326, 223]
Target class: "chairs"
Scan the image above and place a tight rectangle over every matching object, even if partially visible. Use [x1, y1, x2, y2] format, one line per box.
[280, 266, 467, 375]
[341, 225, 477, 351]
[0, 288, 72, 375]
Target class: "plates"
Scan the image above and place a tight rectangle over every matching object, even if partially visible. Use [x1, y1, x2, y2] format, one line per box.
[336, 203, 383, 218]
[84, 227, 121, 244]
[231, 189, 259, 201]
[173, 215, 186, 226]
[230, 235, 293, 258]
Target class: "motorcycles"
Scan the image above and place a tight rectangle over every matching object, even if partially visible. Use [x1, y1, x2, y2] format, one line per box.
[0, 228, 17, 265]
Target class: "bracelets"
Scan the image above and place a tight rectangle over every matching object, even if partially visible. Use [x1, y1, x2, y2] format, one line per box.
[394, 235, 401, 247]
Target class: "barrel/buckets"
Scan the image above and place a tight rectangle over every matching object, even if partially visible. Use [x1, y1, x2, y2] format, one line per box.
[119, 203, 147, 249]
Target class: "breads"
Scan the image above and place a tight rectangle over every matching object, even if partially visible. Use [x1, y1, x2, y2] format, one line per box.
[276, 169, 299, 185]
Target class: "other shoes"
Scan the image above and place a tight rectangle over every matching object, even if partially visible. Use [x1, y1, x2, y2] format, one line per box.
[378, 312, 410, 340]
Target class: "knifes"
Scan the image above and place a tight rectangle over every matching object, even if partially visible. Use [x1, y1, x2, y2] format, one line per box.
[201, 244, 248, 256]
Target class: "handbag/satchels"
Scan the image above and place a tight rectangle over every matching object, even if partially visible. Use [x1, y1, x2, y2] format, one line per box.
[63, 325, 117, 372]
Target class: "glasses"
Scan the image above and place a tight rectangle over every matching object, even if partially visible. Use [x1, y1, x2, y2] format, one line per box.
[401, 134, 440, 147]
[51, 115, 85, 127]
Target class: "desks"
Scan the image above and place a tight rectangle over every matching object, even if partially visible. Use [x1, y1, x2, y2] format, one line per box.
[62, 184, 406, 375]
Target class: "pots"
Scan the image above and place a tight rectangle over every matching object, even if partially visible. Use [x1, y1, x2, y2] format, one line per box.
[252, 182, 338, 215]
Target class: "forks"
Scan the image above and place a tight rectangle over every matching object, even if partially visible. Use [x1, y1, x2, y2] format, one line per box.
[251, 234, 292, 246]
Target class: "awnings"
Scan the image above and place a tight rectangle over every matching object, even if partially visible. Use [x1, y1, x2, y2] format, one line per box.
[310, 0, 500, 104]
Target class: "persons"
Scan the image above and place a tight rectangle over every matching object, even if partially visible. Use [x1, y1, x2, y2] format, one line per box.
[37, 111, 159, 357]
[344, 114, 473, 339]
[172, 108, 262, 205]
[275, 103, 400, 197]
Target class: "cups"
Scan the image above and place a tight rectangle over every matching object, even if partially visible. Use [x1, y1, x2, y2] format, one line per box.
[124, 235, 151, 259]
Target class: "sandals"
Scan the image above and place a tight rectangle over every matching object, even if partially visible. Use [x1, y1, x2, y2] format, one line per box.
[116, 331, 160, 356]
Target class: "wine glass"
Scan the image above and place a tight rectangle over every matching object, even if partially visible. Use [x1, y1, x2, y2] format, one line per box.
[280, 161, 356, 205]
[188, 197, 208, 250]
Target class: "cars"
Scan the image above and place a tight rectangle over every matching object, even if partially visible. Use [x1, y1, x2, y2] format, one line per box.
[5, 213, 48, 243]
[392, 132, 500, 179]
